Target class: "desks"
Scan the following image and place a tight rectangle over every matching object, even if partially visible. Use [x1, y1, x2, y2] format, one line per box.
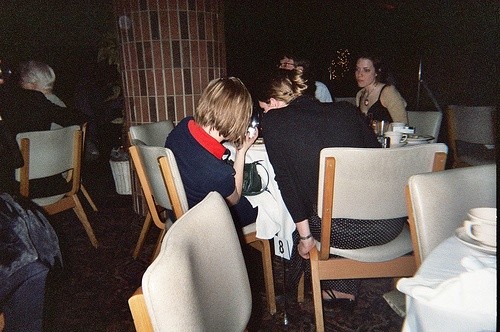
[401, 236, 496, 332]
[221, 136, 435, 243]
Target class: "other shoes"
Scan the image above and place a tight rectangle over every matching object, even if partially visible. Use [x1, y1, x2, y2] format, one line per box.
[307, 290, 356, 307]
[255, 290, 315, 327]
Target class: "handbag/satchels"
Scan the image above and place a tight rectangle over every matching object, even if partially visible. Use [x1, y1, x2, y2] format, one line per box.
[226, 160, 269, 196]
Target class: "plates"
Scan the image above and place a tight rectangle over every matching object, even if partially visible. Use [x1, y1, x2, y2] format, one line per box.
[401, 135, 436, 141]
[452, 227, 497, 255]
[382, 143, 407, 147]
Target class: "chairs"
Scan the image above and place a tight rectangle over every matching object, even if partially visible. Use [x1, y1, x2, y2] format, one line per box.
[127, 102, 496, 332]
[14, 121, 100, 249]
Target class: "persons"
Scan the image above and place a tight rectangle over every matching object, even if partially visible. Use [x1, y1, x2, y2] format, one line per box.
[166, 75, 290, 297]
[256, 50, 408, 308]
[0, 61, 86, 196]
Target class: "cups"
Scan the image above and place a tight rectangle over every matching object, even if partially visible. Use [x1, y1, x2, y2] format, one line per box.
[376, 120, 389, 136]
[384, 131, 408, 144]
[463, 207, 497, 246]
[390, 122, 407, 131]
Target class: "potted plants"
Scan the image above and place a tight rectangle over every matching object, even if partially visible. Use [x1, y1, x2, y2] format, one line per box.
[95, 29, 132, 196]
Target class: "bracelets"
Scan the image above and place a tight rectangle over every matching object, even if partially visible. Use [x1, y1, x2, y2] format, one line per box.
[300, 234, 313, 240]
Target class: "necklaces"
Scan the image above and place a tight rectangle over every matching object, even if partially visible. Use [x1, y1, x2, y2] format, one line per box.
[364, 82, 379, 106]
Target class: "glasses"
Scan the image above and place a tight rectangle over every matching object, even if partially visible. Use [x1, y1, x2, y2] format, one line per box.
[275, 62, 296, 69]
